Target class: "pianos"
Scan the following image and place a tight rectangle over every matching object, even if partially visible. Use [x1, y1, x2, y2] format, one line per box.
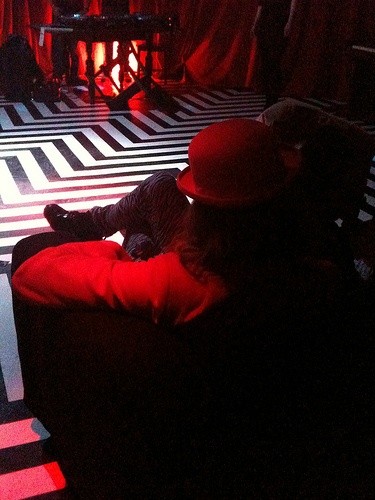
[60, 7, 180, 105]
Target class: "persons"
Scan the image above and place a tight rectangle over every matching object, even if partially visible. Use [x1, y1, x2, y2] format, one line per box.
[10, 118, 374, 325]
[250, 0, 296, 113]
[49, 0, 85, 86]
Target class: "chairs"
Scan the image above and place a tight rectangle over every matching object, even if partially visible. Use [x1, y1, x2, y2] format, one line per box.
[12, 97, 374, 438]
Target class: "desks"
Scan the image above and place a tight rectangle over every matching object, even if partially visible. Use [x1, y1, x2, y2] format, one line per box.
[64, 13, 178, 106]
